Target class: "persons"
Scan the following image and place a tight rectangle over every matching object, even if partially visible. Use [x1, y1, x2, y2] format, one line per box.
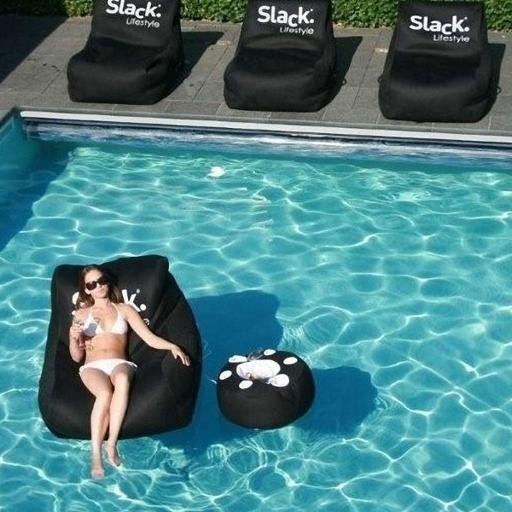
[67, 264, 192, 480]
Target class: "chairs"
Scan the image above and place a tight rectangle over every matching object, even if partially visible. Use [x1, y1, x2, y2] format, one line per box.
[38, 254, 202, 438]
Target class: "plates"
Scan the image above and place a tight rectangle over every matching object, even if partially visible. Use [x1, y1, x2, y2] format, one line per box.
[220, 350, 298, 389]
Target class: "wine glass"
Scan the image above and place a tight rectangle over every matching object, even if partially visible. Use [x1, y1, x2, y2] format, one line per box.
[73, 319, 88, 350]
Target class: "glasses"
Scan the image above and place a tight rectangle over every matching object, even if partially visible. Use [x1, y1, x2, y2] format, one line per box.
[85, 277, 107, 291]
